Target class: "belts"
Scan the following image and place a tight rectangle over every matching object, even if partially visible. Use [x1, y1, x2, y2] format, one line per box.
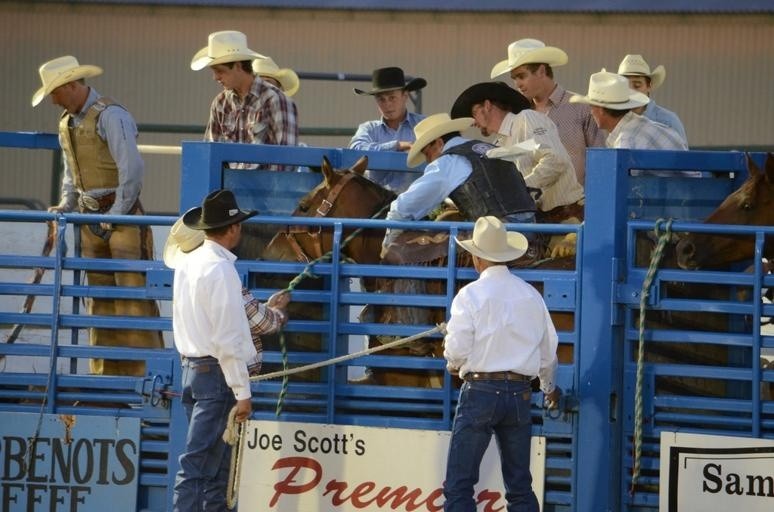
[80, 192, 117, 212]
[471, 372, 524, 382]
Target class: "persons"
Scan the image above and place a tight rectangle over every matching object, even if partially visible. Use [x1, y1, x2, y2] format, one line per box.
[249, 54, 297, 102]
[443, 210, 562, 511]
[162, 206, 289, 371]
[345, 65, 427, 194]
[379, 111, 538, 267]
[448, 79, 583, 221]
[190, 27, 299, 174]
[171, 188, 257, 512]
[28, 54, 164, 376]
[566, 67, 690, 152]
[489, 39, 605, 184]
[613, 53, 684, 138]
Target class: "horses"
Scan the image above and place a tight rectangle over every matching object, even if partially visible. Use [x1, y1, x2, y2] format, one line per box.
[259, 153, 728, 418]
[675, 150, 774, 274]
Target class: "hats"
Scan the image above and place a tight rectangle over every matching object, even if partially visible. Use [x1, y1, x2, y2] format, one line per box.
[454, 215, 529, 264]
[250, 58, 299, 98]
[162, 190, 260, 269]
[450, 81, 532, 120]
[603, 53, 666, 91]
[191, 31, 266, 72]
[490, 39, 568, 79]
[570, 70, 652, 111]
[32, 55, 103, 107]
[352, 67, 427, 96]
[406, 113, 476, 169]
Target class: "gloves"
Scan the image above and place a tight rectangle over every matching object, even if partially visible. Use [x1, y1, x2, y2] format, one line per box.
[379, 228, 402, 260]
[551, 232, 575, 259]
